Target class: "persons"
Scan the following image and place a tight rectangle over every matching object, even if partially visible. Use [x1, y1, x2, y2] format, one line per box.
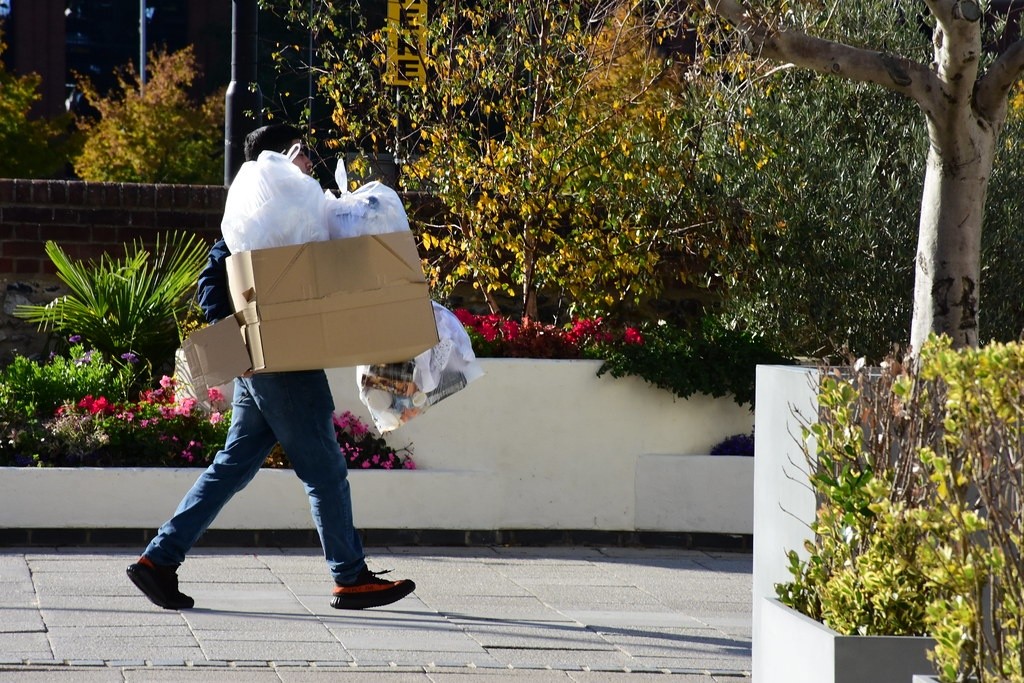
[126, 124, 416, 610]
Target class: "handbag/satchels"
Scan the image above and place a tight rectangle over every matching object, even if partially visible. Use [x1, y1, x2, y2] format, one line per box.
[220, 143, 330, 254]
[355, 300, 486, 435]
[324, 157, 411, 240]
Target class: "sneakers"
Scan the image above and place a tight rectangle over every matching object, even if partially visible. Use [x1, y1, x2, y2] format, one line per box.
[126, 555, 195, 610]
[330, 569, 416, 610]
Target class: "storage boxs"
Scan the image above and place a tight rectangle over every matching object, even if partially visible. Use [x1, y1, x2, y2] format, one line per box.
[225, 230, 442, 374]
